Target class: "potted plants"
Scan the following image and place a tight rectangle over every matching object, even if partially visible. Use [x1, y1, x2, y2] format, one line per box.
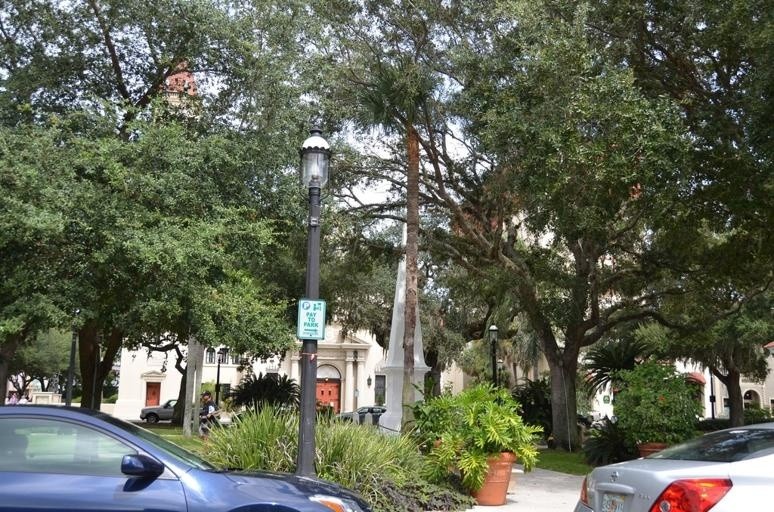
[615, 359, 703, 460]
[422, 380, 538, 507]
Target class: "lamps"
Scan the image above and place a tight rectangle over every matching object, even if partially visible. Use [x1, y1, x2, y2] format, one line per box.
[367, 373, 371, 389]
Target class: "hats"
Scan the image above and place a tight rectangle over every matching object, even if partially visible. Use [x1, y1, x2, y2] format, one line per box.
[201, 390, 211, 395]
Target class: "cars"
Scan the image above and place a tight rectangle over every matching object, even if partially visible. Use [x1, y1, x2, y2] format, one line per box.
[573, 421, 773, 512]
[580, 410, 616, 429]
[141, 399, 388, 436]
[0, 404, 374, 512]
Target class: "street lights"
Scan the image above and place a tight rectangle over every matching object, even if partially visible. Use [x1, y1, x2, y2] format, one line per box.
[215, 348, 223, 406]
[65, 311, 84, 406]
[488, 319, 498, 390]
[708, 358, 714, 421]
[293, 128, 333, 478]
[496, 356, 504, 389]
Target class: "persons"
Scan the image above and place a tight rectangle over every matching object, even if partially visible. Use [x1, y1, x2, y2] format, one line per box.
[198, 391, 221, 438]
